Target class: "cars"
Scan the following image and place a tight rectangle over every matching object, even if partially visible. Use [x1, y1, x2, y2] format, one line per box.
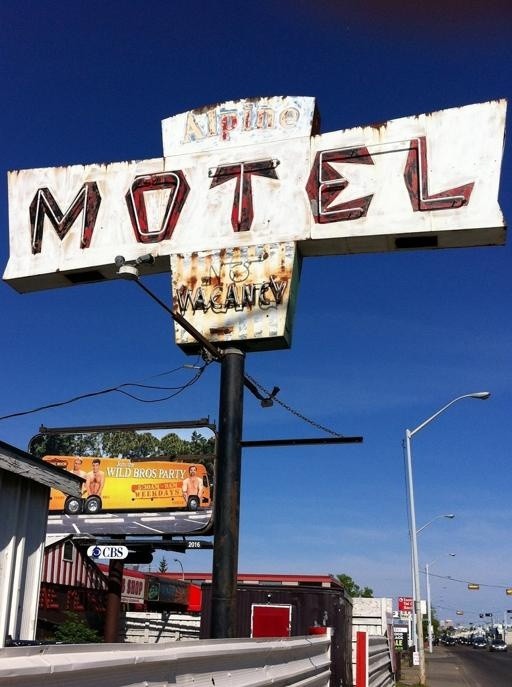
[489, 638, 507, 652]
[425, 634, 439, 645]
[441, 635, 472, 646]
[473, 636, 486, 648]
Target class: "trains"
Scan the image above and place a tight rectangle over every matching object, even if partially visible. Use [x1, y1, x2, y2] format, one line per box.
[92, 562, 202, 616]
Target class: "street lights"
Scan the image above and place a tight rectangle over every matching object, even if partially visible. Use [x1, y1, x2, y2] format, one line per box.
[425, 552, 456, 653]
[409, 513, 456, 654]
[405, 388, 490, 685]
[174, 558, 185, 582]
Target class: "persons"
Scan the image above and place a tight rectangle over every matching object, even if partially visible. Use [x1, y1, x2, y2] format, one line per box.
[435, 636, 439, 645]
[182, 466, 203, 507]
[85, 459, 105, 498]
[61, 459, 87, 516]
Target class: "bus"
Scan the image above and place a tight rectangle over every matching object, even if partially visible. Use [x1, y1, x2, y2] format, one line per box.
[40, 454, 211, 514]
